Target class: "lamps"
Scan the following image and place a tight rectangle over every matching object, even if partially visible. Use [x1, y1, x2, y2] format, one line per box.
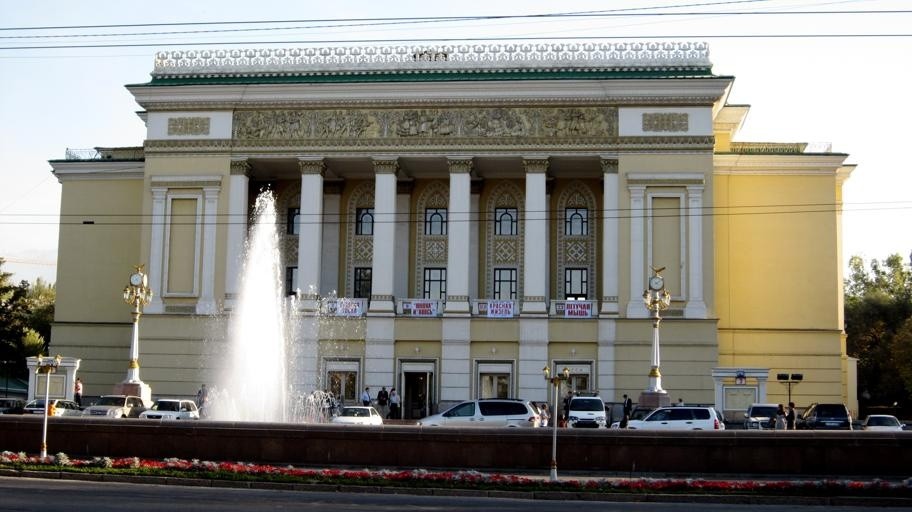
[735, 370, 746, 385]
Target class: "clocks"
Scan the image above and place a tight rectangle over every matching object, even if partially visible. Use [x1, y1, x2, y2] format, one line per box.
[130, 273, 142, 285]
[650, 277, 664, 290]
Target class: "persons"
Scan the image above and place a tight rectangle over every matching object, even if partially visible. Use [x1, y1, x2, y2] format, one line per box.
[362, 388, 371, 406]
[559, 390, 572, 429]
[531, 402, 551, 427]
[378, 386, 400, 419]
[775, 402, 797, 430]
[76, 378, 82, 406]
[197, 384, 207, 408]
[620, 395, 632, 429]
[677, 398, 685, 406]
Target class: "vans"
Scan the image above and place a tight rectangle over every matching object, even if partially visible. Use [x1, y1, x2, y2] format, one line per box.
[565, 391, 609, 428]
[416, 396, 540, 427]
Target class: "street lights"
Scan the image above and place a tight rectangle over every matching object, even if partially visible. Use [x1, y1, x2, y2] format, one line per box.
[542, 364, 570, 478]
[34, 353, 63, 462]
[640, 289, 672, 394]
[122, 285, 152, 382]
[775, 373, 803, 405]
[3, 359, 17, 397]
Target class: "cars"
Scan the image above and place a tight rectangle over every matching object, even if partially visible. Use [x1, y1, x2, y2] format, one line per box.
[333, 404, 384, 427]
[861, 414, 907, 432]
[0, 397, 25, 413]
[23, 398, 83, 417]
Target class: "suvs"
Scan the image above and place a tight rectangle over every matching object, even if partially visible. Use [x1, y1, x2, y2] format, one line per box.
[611, 406, 726, 430]
[742, 403, 783, 430]
[798, 401, 853, 430]
[83, 395, 146, 419]
[139, 398, 200, 421]
